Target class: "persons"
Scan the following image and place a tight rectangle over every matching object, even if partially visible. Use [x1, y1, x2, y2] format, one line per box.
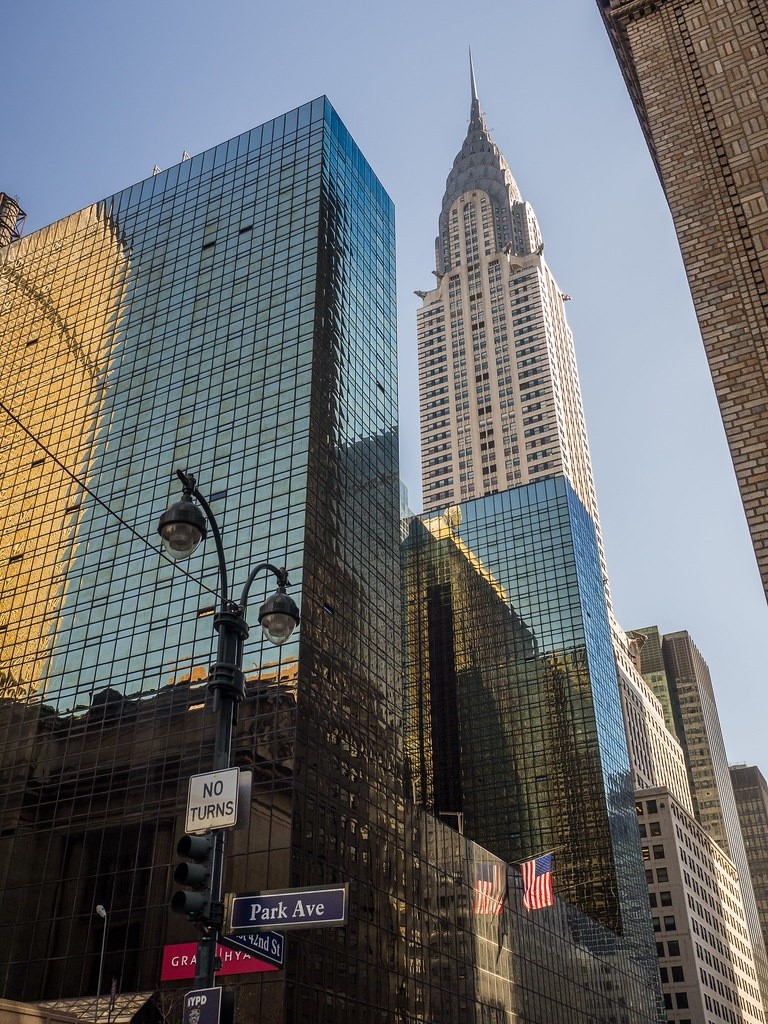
[519, 851, 556, 914]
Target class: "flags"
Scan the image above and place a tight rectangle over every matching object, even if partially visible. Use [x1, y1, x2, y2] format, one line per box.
[473, 863, 505, 915]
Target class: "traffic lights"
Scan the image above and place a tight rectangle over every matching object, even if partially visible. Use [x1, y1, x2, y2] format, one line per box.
[171, 831, 215, 920]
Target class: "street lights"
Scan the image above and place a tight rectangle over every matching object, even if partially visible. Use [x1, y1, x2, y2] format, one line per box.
[95, 905, 107, 1024]
[159, 469, 302, 1024]
